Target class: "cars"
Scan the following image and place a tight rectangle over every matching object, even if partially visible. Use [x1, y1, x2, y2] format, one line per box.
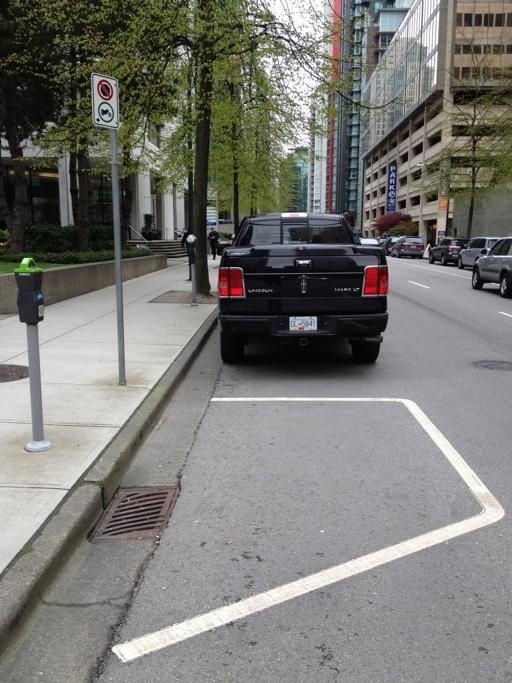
[359, 234, 512, 298]
[217, 212, 388, 363]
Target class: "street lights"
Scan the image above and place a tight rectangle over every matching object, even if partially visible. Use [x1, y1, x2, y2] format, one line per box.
[416, 164, 450, 236]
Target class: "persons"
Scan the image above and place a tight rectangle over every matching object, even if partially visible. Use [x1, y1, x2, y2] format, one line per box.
[208, 225, 220, 259]
[179, 227, 190, 249]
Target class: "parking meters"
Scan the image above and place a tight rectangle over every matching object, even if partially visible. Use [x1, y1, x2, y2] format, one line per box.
[14, 258, 51, 452]
